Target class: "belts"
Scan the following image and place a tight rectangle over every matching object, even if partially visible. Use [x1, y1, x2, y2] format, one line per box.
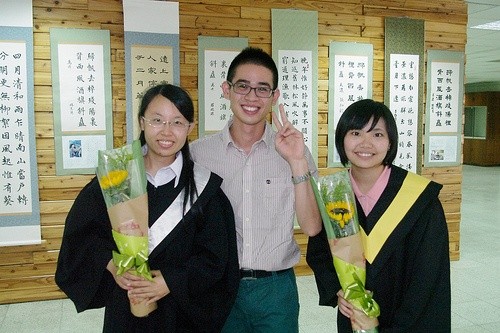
[240, 269, 287, 279]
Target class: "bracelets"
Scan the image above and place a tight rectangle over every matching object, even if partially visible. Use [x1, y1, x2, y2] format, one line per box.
[292, 171, 311, 184]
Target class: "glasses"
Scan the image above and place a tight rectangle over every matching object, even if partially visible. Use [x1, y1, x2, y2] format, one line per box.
[228, 81, 274, 98]
[142, 115, 189, 130]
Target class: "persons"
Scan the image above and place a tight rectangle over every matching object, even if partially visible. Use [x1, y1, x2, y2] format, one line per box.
[305, 99, 452, 333]
[54, 84, 241, 333]
[139, 45, 322, 333]
